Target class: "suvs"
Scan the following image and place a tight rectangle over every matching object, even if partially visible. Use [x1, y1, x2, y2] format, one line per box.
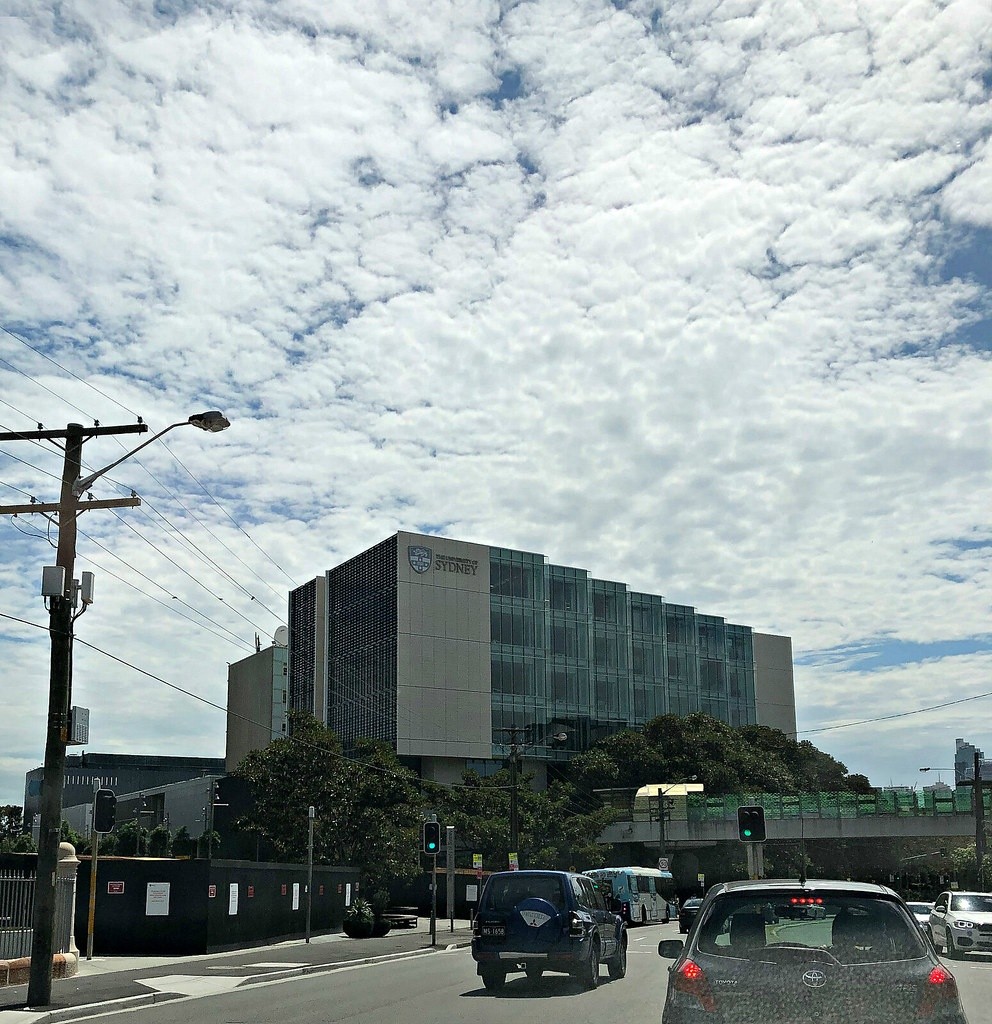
[927, 890, 992, 959]
[472, 867, 629, 988]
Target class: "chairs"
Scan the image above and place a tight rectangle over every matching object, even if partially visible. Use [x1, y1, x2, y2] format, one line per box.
[718, 912, 770, 951]
[829, 914, 895, 965]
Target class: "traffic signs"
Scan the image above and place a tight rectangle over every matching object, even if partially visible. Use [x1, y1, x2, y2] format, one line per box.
[107, 881, 124, 894]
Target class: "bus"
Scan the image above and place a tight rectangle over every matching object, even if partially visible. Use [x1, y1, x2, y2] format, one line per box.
[585, 866, 672, 925]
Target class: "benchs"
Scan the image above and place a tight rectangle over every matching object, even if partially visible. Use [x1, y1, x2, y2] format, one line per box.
[382, 914, 418, 929]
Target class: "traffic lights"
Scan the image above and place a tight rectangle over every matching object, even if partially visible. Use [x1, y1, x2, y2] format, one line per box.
[737, 806, 767, 842]
[940, 847, 947, 858]
[422, 821, 441, 854]
[92, 788, 117, 833]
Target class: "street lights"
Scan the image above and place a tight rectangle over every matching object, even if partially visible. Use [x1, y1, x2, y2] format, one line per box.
[494, 723, 568, 872]
[650, 775, 698, 869]
[919, 751, 984, 892]
[195, 781, 230, 858]
[132, 793, 168, 857]
[0, 409, 234, 1004]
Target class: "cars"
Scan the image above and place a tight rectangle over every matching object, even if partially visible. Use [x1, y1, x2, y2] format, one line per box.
[679, 899, 704, 933]
[660, 876, 970, 1024]
[906, 900, 935, 933]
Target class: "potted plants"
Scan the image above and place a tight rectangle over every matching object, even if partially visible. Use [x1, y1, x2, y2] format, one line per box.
[342, 898, 375, 939]
[369, 890, 391, 937]
